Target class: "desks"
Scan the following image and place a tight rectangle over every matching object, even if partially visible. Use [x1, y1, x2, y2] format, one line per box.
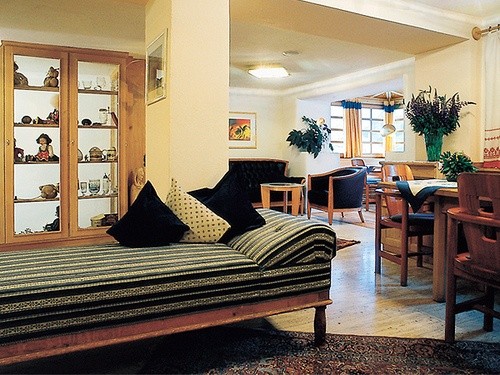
[259, 182, 301, 216]
[289, 158, 341, 177]
[378, 161, 443, 183]
[377, 180, 493, 285]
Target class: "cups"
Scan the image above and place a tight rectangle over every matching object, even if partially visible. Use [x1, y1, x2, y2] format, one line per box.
[82, 80, 93, 90]
[89, 179, 101, 196]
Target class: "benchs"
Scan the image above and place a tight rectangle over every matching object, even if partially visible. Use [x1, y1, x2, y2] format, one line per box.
[229, 159, 306, 217]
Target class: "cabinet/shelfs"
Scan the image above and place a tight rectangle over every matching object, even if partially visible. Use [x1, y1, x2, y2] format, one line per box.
[0, 40, 146, 250]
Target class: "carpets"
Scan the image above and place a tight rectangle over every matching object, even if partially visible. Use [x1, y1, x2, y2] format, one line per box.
[136, 327, 500, 375]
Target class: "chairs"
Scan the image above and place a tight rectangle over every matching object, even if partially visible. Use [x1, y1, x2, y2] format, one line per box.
[307, 165, 368, 224]
[351, 159, 499, 343]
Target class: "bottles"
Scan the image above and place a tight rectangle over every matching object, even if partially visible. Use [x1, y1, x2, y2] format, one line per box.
[103, 175, 111, 194]
[89, 146, 117, 162]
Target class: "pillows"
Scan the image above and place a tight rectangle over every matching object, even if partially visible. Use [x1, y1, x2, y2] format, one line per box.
[106, 164, 266, 246]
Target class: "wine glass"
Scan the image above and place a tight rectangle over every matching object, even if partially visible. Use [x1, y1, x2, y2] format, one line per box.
[81, 182, 87, 197]
[97, 77, 106, 90]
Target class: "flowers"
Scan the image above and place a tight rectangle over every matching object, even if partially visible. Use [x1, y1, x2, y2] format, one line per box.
[287, 117, 334, 157]
[404, 89, 460, 136]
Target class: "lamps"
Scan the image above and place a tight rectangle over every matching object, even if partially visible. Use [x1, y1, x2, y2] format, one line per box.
[249, 63, 288, 79]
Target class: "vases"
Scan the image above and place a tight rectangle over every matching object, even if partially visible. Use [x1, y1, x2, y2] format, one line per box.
[424, 134, 442, 162]
[301, 151, 317, 159]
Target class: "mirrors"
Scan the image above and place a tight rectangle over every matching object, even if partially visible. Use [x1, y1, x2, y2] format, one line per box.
[329, 90, 406, 157]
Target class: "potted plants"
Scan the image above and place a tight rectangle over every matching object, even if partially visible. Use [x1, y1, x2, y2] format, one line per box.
[439, 152, 474, 183]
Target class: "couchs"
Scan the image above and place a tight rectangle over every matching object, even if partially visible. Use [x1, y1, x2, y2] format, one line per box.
[0, 209, 336, 375]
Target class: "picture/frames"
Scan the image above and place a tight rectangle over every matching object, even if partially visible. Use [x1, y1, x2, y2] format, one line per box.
[146, 28, 167, 105]
[229, 112, 257, 149]
